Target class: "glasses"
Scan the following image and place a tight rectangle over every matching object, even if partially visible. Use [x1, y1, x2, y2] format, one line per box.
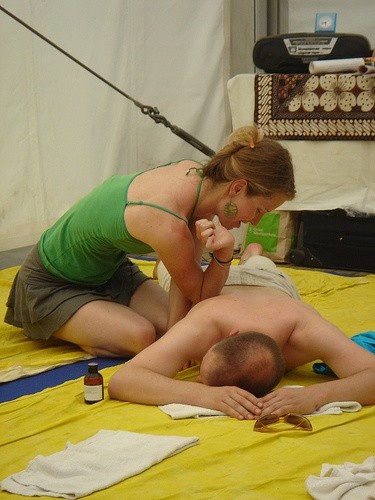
[253, 412, 313, 433]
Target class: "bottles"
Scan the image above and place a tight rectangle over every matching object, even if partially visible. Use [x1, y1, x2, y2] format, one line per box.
[84, 362, 104, 405]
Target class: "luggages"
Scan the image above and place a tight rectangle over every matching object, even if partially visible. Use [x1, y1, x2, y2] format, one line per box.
[287, 208, 375, 272]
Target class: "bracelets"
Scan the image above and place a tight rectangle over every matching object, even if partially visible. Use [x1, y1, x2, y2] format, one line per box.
[213, 252, 234, 268]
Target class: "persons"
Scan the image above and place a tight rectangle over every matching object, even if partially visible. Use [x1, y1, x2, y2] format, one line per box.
[107, 242, 375, 422]
[4, 123, 297, 357]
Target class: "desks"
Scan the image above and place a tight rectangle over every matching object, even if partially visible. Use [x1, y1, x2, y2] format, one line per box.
[226, 72, 375, 252]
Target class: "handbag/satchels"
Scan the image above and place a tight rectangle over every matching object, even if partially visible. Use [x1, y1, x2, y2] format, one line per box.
[208, 210, 293, 263]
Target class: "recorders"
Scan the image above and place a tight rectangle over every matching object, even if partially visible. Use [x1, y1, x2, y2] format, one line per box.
[252, 30, 370, 75]
